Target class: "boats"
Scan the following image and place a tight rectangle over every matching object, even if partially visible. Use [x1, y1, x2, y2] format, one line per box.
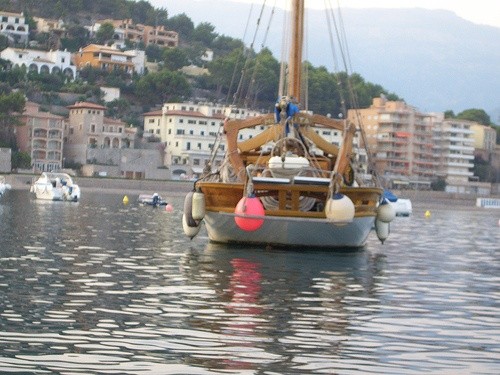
[138, 193, 168, 207]
[31, 171, 81, 202]
[380, 189, 413, 217]
[0, 176, 7, 198]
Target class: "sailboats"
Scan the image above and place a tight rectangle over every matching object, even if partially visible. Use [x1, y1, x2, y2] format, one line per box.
[191, 1, 383, 250]
